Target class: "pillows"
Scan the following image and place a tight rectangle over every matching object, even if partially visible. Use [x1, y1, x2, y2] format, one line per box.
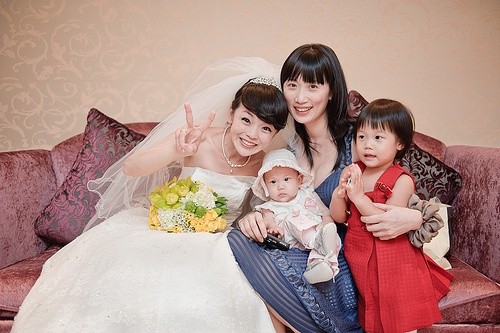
[346, 90, 463, 205]
[34, 108, 182, 244]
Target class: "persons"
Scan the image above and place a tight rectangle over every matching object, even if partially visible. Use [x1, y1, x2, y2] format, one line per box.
[228, 43, 424, 333]
[329, 98, 418, 333]
[76, 77, 290, 333]
[253, 147, 342, 282]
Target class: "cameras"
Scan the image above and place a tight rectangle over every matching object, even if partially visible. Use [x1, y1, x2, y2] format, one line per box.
[263, 233, 289, 251]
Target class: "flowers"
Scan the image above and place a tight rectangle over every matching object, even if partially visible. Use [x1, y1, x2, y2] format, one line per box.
[148, 176, 229, 234]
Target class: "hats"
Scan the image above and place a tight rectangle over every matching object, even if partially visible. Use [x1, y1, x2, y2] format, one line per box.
[252, 149, 312, 201]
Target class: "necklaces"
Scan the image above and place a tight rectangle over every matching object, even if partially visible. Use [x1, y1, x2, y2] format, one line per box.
[222, 126, 252, 174]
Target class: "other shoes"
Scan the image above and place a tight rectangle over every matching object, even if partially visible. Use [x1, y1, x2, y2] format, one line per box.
[315, 223, 337, 256]
[303, 259, 334, 284]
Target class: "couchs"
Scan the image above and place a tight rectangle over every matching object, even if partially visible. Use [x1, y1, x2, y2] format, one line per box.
[0, 122, 500, 333]
